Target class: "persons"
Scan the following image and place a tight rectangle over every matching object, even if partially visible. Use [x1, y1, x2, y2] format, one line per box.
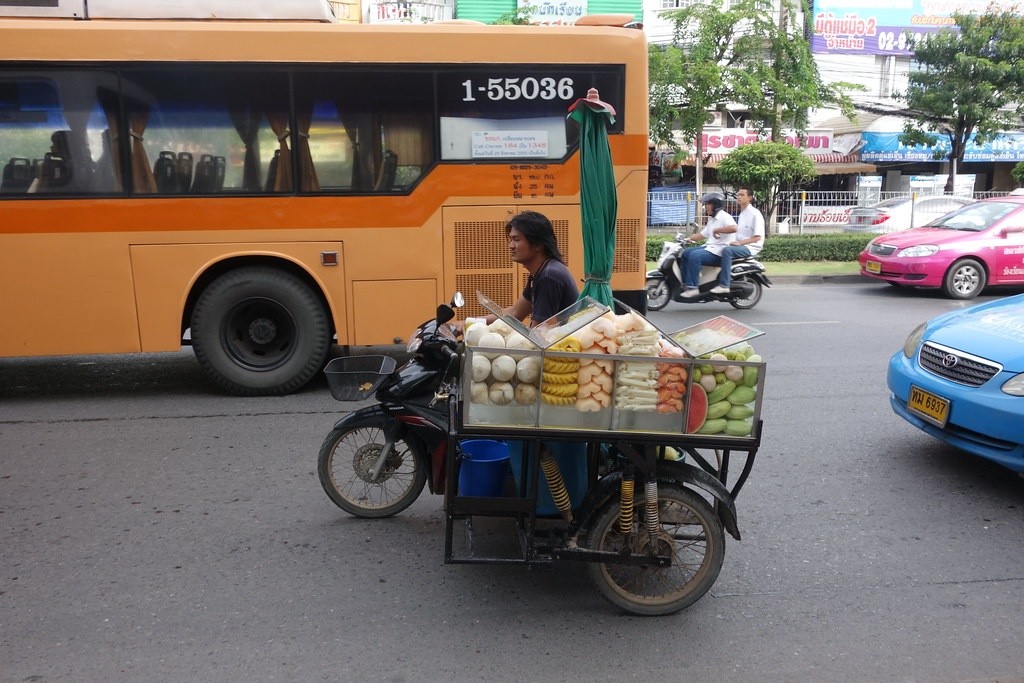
[27, 130, 73, 192]
[89, 129, 115, 191]
[709, 187, 765, 293]
[680, 193, 738, 297]
[447, 211, 581, 335]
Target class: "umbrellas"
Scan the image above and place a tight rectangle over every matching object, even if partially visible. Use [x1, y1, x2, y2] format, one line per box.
[568, 88, 619, 315]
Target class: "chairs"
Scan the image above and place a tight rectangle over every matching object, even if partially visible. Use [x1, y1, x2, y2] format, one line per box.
[153, 151, 176, 194]
[264, 150, 281, 192]
[32, 159, 43, 179]
[212, 156, 226, 193]
[37, 152, 71, 192]
[176, 152, 193, 193]
[0, 158, 32, 194]
[190, 154, 215, 193]
[373, 149, 398, 191]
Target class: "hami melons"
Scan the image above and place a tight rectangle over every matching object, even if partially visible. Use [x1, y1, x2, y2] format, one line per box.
[575, 306, 645, 412]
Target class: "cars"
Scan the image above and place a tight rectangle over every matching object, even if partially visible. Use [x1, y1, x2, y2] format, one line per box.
[841, 191, 982, 235]
[857, 188, 1024, 300]
[887, 292, 1024, 474]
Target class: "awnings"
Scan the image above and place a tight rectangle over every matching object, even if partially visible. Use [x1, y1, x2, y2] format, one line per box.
[681, 160, 876, 175]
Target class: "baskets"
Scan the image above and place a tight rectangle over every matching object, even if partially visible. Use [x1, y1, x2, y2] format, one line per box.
[323, 354, 397, 402]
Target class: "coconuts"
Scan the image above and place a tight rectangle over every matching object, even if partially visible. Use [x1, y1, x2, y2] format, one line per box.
[464, 318, 540, 406]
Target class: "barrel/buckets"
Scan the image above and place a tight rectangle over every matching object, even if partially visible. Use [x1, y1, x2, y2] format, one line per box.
[664, 446, 686, 484]
[457, 438, 512, 497]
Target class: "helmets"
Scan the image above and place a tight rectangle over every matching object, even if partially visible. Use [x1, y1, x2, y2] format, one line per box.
[699, 193, 727, 217]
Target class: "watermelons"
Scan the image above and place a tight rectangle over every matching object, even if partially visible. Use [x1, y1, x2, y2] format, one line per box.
[686, 382, 708, 434]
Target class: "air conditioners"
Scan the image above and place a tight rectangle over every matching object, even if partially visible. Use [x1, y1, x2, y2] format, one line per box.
[744, 119, 764, 130]
[702, 111, 723, 127]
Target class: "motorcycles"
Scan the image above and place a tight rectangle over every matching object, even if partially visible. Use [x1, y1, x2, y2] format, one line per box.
[317, 292, 766, 616]
[645, 223, 772, 311]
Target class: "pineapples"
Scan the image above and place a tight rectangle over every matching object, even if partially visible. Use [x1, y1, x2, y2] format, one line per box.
[541, 336, 582, 406]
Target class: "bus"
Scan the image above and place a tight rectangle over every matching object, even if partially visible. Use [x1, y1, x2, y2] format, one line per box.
[0, 0, 651, 398]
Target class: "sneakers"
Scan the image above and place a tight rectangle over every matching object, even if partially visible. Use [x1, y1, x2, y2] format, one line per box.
[680, 288, 700, 298]
[710, 285, 732, 294]
